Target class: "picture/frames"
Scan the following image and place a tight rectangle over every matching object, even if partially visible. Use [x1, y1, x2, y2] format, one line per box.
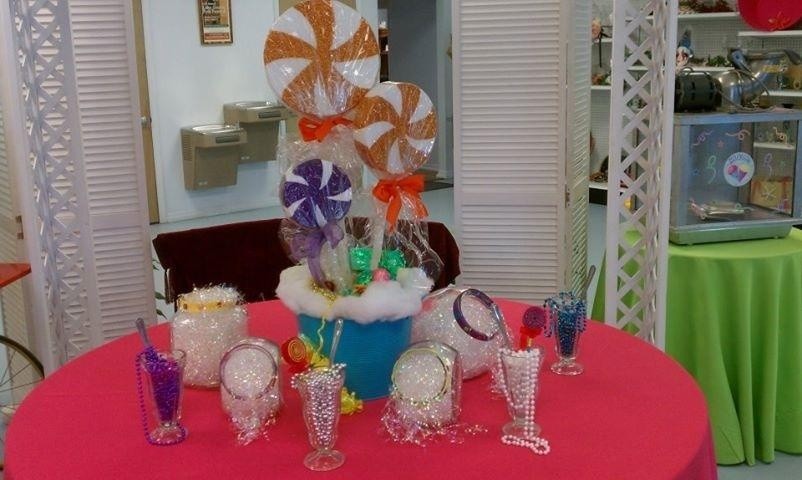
[198, 0, 233, 45]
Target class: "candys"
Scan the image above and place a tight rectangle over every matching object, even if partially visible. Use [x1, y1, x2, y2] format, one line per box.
[264, 0, 381, 243]
[353, 79, 437, 271]
[281, 158, 356, 293]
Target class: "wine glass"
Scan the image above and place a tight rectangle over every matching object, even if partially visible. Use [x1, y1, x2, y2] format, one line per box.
[499, 342, 543, 439]
[294, 368, 345, 472]
[550, 295, 587, 377]
[138, 349, 188, 445]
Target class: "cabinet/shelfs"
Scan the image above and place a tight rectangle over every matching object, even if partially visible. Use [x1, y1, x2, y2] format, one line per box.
[589, 12, 802, 207]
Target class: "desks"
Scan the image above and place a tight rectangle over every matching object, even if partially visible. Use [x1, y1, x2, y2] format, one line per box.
[617, 219, 802, 465]
[3, 293, 709, 480]
